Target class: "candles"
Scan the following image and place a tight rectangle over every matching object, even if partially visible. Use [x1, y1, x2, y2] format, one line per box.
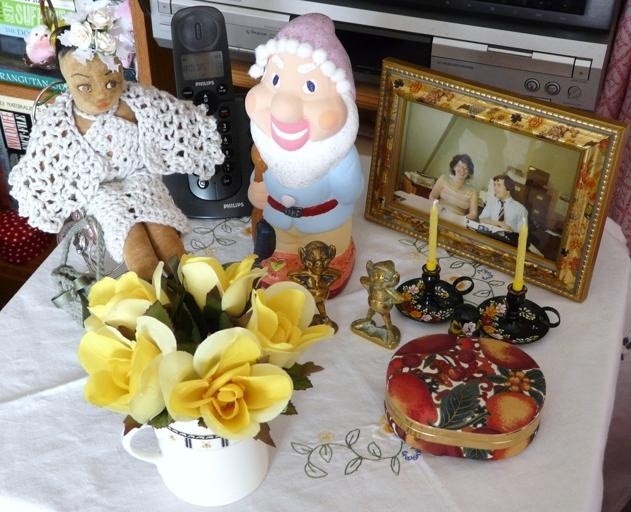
[427, 200, 439, 271]
[513, 215, 529, 291]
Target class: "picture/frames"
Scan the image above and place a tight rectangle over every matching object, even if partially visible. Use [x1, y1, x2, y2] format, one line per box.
[364, 59, 630, 302]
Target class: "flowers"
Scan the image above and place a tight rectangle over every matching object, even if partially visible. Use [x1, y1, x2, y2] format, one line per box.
[78, 222, 335, 448]
[57, 0, 136, 73]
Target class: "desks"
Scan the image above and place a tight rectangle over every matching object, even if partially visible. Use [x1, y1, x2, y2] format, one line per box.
[0, 153, 631, 512]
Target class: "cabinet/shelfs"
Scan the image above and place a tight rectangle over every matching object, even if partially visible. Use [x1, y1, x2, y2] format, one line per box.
[0, 0, 607, 204]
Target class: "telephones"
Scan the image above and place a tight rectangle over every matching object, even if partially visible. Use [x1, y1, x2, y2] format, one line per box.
[162, 6, 255, 220]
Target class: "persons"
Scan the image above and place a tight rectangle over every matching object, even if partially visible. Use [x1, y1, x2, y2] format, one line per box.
[5, 2, 230, 278]
[242, 10, 371, 297]
[478, 173, 530, 243]
[426, 153, 480, 222]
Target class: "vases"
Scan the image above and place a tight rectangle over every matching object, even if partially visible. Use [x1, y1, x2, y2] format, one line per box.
[121, 419, 270, 508]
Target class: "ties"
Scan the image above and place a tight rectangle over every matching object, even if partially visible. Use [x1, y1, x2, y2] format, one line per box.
[499, 200, 506, 222]
